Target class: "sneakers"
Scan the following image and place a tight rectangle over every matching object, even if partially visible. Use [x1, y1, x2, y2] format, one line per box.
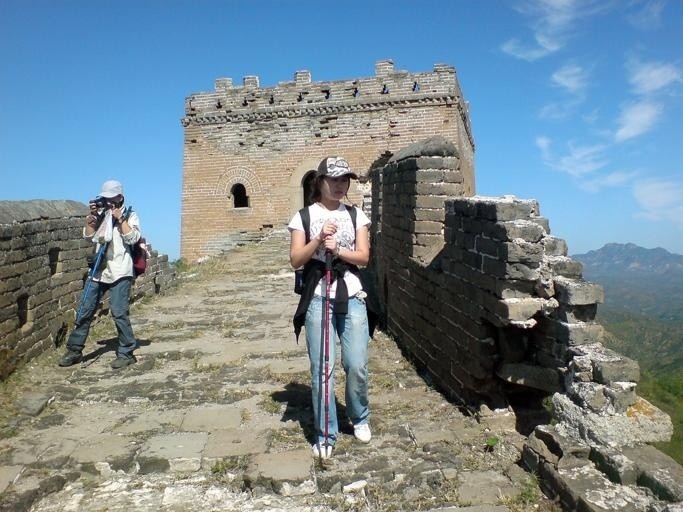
[112, 355, 137, 368]
[59, 350, 83, 366]
[352, 422, 371, 443]
[312, 442, 332, 458]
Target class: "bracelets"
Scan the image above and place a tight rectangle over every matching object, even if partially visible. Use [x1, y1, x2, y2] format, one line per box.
[336, 248, 340, 256]
[115, 215, 125, 225]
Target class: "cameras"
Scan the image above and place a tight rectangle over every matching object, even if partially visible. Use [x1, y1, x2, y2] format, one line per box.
[95, 198, 107, 208]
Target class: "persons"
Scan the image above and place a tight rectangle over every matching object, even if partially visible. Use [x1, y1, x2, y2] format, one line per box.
[287, 156, 371, 457]
[58, 180, 142, 368]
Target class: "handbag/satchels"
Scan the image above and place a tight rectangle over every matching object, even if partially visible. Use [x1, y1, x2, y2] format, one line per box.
[124, 210, 146, 277]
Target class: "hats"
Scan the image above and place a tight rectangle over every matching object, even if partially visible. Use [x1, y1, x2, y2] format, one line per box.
[100, 181, 123, 198]
[317, 157, 358, 181]
